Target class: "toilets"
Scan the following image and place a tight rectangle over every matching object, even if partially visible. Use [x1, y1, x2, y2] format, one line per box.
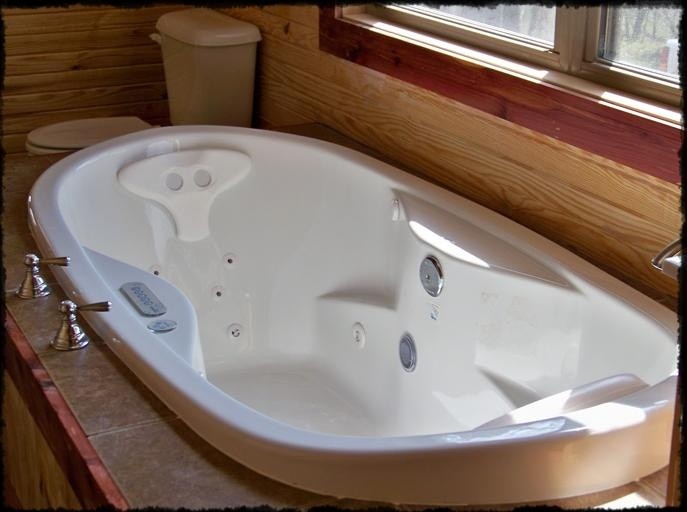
[23, 7, 263, 156]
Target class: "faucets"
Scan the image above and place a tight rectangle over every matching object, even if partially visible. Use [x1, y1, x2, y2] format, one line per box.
[650, 241, 683, 270]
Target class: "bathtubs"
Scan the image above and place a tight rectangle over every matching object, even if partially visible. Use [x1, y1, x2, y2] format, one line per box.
[28, 124, 679, 505]
